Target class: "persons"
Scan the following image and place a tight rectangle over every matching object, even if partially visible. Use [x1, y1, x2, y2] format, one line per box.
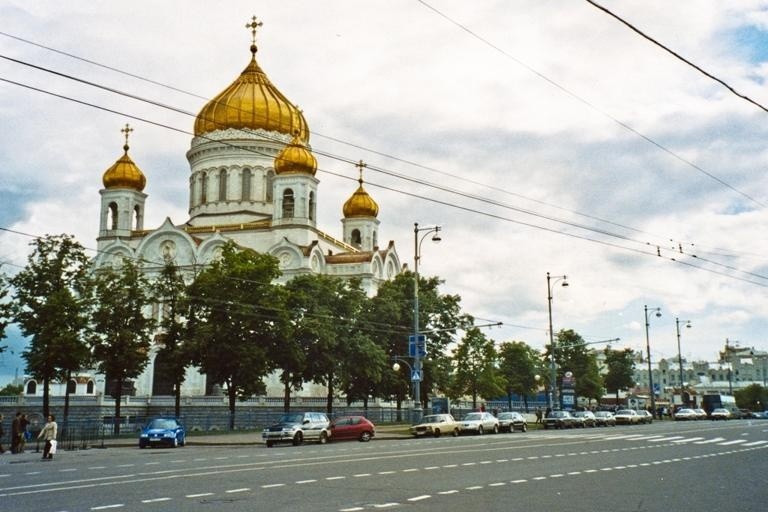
[0, 414, 7, 454]
[17, 414, 31, 453]
[12, 412, 23, 454]
[479, 402, 684, 425]
[37, 415, 58, 461]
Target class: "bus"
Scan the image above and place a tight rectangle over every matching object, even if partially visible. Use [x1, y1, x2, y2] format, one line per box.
[701, 395, 736, 412]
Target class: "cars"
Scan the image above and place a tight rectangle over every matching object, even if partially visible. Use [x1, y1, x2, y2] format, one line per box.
[541, 406, 768, 430]
[140, 417, 186, 448]
[409, 411, 526, 438]
[330, 415, 376, 441]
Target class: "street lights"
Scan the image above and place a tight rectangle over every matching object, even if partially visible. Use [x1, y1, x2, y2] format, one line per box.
[643, 304, 662, 418]
[392, 352, 422, 424]
[544, 272, 567, 410]
[725, 338, 739, 394]
[676, 318, 691, 402]
[411, 223, 442, 426]
[535, 373, 557, 410]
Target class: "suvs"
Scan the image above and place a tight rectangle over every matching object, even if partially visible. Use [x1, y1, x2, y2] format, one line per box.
[262, 412, 331, 447]
